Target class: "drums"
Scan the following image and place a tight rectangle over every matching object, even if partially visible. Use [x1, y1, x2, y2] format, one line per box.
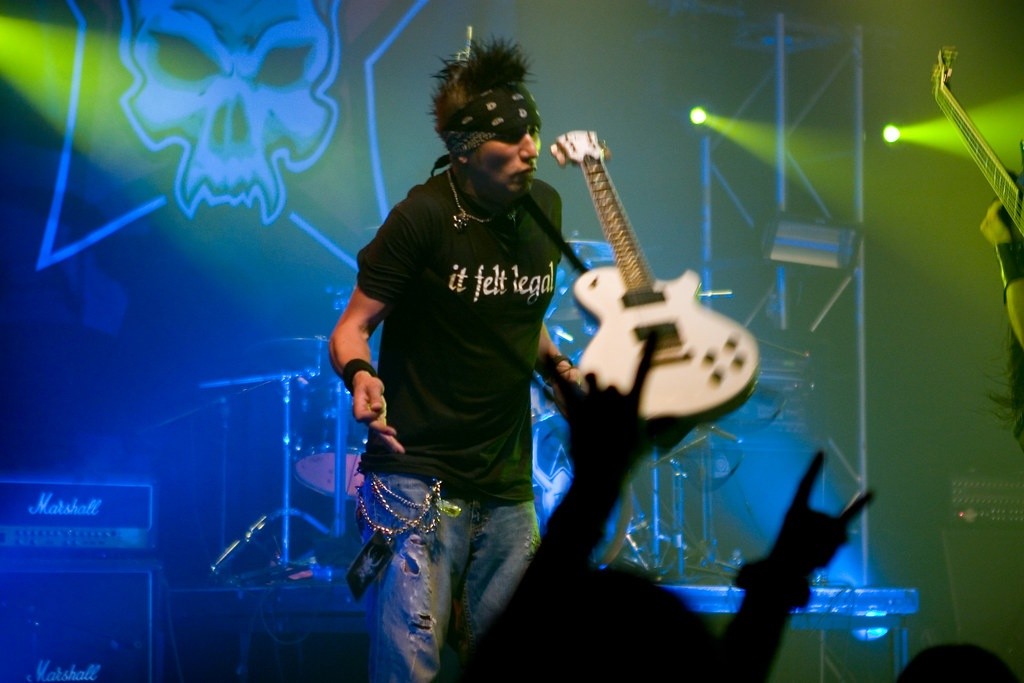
[291, 375, 370, 503]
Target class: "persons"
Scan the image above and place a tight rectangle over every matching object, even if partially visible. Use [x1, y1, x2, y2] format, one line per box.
[327, 36, 580, 683]
[467, 176, 1024, 683]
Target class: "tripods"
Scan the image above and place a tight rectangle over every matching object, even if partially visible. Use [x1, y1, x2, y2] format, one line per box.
[208, 336, 333, 585]
[623, 420, 730, 587]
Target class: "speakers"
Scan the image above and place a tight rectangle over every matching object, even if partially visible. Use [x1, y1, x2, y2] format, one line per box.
[941, 525, 1024, 679]
[0, 556, 164, 681]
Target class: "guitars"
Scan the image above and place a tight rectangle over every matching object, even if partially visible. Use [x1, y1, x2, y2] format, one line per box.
[928, 45, 1024, 238]
[549, 128, 761, 428]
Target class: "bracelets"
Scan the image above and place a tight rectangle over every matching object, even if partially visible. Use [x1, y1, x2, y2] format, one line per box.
[731, 564, 815, 607]
[542, 354, 572, 386]
[343, 359, 377, 394]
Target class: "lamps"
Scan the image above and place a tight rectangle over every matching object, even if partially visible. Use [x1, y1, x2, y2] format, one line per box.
[756, 212, 863, 275]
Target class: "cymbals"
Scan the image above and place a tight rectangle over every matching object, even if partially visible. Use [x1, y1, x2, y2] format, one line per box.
[251, 335, 333, 354]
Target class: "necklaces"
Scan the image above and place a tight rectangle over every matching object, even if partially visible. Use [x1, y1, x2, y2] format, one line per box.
[448, 166, 498, 230]
